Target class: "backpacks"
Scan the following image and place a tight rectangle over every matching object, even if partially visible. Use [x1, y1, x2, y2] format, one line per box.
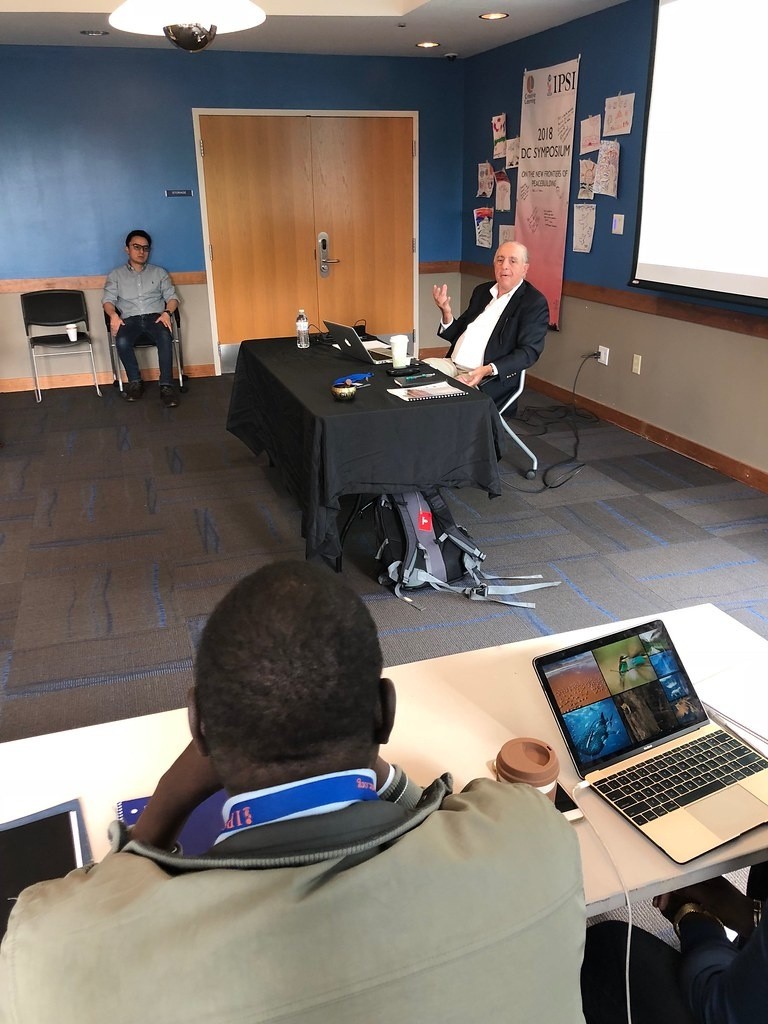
[368, 488, 562, 611]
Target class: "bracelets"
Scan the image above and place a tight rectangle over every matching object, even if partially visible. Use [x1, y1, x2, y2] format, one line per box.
[752, 899, 761, 928]
[164, 310, 172, 316]
[489, 364, 494, 376]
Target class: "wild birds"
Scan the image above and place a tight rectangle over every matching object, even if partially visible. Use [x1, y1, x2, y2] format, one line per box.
[618, 655, 627, 688]
[629, 651, 648, 668]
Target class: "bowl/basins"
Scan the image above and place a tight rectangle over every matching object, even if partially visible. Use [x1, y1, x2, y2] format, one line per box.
[331, 384, 357, 400]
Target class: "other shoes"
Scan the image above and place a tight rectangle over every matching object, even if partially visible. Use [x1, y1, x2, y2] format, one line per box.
[124, 380, 145, 401]
[160, 385, 179, 407]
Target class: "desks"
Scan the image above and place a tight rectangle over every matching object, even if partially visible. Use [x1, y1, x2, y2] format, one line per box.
[1, 603, 767, 1024]
[225, 331, 506, 569]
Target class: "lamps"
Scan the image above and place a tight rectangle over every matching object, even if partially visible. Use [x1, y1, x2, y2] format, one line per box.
[109, 0, 266, 53]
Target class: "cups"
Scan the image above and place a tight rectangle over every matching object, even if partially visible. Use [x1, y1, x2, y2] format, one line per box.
[65, 324, 77, 342]
[495, 739, 561, 806]
[390, 335, 409, 369]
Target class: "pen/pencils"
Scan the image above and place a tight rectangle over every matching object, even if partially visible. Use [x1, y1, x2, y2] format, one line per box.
[406, 373, 435, 380]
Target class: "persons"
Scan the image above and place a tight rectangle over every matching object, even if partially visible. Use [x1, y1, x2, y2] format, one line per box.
[101, 230, 181, 408]
[0, 559, 588, 1024]
[581, 862, 768, 1024]
[421, 241, 550, 400]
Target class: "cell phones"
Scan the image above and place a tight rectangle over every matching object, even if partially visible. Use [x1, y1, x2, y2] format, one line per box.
[494, 760, 586, 824]
[387, 366, 420, 377]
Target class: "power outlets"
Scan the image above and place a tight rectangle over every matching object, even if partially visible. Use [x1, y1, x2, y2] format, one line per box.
[596, 345, 609, 365]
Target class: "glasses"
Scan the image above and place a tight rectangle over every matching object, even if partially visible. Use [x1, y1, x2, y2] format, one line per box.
[128, 245, 151, 252]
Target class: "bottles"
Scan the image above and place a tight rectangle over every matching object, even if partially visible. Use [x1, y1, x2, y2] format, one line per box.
[296, 309, 310, 349]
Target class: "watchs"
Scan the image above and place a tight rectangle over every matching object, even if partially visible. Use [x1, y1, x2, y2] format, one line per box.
[673, 903, 722, 938]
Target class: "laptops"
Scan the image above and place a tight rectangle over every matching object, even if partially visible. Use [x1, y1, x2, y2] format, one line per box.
[531, 619, 768, 865]
[323, 319, 414, 364]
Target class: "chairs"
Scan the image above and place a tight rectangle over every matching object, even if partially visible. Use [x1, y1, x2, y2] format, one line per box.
[20, 290, 102, 403]
[445, 343, 539, 476]
[104, 304, 184, 398]
